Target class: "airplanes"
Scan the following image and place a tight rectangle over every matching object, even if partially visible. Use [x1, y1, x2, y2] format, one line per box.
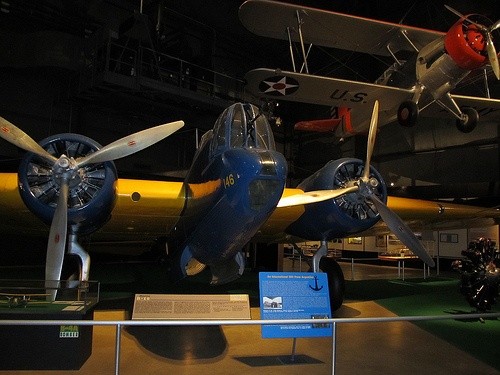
[239, 0, 499, 133]
[4, 98, 497, 309]
